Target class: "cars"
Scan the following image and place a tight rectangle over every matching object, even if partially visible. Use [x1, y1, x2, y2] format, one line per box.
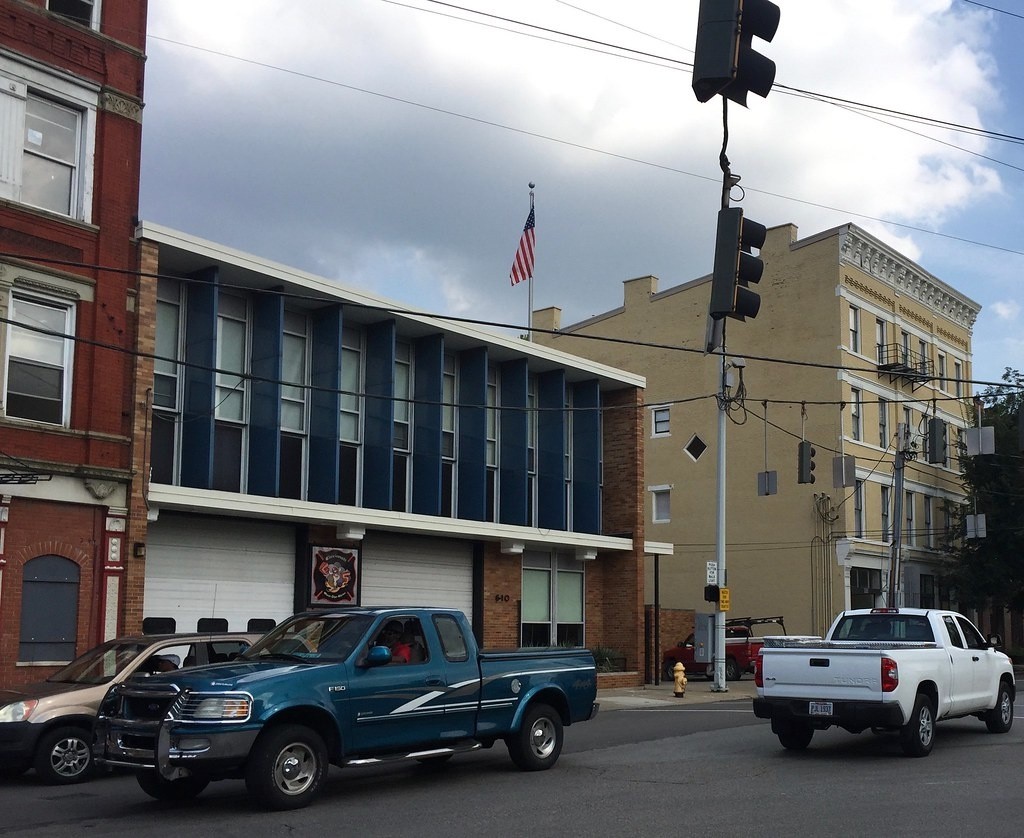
[0, 632, 318, 787]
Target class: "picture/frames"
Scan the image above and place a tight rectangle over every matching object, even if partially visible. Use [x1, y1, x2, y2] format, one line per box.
[307, 543, 361, 608]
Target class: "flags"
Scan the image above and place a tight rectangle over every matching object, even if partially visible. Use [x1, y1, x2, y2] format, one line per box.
[509, 203, 535, 286]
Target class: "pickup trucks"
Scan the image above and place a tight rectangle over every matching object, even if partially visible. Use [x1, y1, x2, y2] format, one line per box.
[663, 616, 822, 681]
[753, 608, 1017, 757]
[90, 605, 601, 812]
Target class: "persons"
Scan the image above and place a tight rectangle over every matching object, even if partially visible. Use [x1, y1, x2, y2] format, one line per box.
[158, 654, 180, 672]
[387, 624, 424, 663]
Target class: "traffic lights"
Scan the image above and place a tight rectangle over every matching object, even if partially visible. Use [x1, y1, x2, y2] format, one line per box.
[799, 442, 816, 484]
[691, 0, 780, 109]
[929, 419, 948, 464]
[710, 207, 767, 322]
[704, 585, 720, 603]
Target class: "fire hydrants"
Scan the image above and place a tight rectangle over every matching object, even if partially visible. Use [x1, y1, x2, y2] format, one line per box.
[673, 662, 687, 698]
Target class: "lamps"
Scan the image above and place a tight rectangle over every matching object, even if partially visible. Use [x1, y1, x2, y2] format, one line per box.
[336, 524, 365, 541]
[501, 540, 526, 555]
[575, 548, 597, 561]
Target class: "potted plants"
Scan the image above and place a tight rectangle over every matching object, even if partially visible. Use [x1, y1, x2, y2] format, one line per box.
[596, 643, 638, 689]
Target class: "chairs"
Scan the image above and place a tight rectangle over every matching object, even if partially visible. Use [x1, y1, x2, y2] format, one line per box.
[212, 652, 227, 662]
[906, 625, 929, 638]
[401, 632, 424, 663]
[183, 656, 195, 666]
[864, 622, 892, 635]
